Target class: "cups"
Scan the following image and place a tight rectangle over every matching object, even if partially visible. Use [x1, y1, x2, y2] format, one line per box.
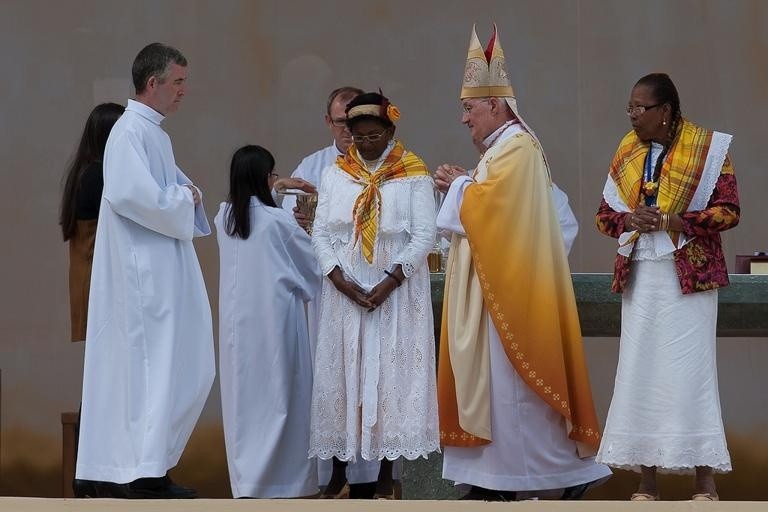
[295, 192, 318, 235]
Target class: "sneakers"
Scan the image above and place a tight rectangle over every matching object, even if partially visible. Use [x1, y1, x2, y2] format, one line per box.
[693, 492, 720, 501]
[631, 490, 660, 501]
[349, 481, 378, 499]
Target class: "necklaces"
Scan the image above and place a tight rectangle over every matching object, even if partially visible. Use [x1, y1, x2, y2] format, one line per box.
[641, 179, 657, 196]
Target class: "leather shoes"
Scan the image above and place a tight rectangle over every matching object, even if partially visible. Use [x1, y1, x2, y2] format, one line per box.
[73, 479, 98, 498]
[123, 475, 197, 499]
[95, 481, 127, 497]
[558, 480, 596, 500]
[459, 485, 516, 501]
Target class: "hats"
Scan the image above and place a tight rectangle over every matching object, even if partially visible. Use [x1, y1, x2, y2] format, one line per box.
[460, 22, 515, 100]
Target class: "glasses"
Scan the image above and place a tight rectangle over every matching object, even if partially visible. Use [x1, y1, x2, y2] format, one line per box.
[271, 174, 279, 180]
[354, 130, 387, 143]
[626, 104, 660, 115]
[464, 100, 488, 113]
[333, 120, 347, 126]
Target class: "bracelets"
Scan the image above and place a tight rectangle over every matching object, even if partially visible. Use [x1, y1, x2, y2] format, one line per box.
[657, 211, 670, 231]
[389, 273, 402, 289]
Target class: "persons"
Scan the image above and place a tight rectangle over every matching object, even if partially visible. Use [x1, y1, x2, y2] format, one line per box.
[591, 72, 742, 500]
[308, 90, 445, 500]
[212, 144, 323, 499]
[433, 88, 612, 498]
[71, 41, 217, 500]
[56, 100, 134, 499]
[280, 86, 364, 240]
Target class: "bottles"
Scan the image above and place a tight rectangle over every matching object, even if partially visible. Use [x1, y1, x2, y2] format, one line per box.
[426, 243, 443, 273]
